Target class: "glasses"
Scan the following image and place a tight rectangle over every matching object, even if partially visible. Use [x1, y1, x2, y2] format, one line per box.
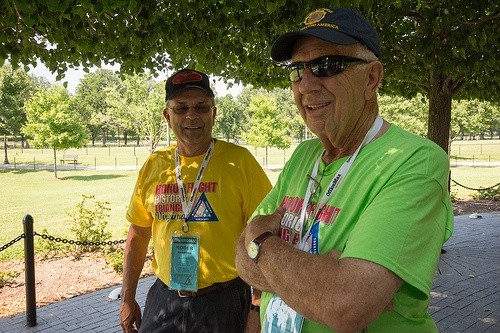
[287, 55, 372, 82]
[165, 103, 214, 115]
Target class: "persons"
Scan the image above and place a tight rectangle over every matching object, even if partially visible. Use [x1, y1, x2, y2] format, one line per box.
[120, 68, 273, 333]
[236, 6, 453, 333]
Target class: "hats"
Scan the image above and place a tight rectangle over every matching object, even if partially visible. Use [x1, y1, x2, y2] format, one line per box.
[271, 7, 381, 62]
[165, 68, 215, 103]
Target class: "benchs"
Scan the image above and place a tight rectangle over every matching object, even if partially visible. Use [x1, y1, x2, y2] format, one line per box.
[59, 154, 79, 165]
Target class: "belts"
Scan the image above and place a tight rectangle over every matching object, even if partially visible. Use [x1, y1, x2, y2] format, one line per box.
[163, 276, 242, 298]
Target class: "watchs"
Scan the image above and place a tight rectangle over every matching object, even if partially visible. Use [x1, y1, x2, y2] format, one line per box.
[246, 232, 272, 263]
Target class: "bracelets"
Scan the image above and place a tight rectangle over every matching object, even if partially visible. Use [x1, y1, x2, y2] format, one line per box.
[250, 304, 261, 312]
